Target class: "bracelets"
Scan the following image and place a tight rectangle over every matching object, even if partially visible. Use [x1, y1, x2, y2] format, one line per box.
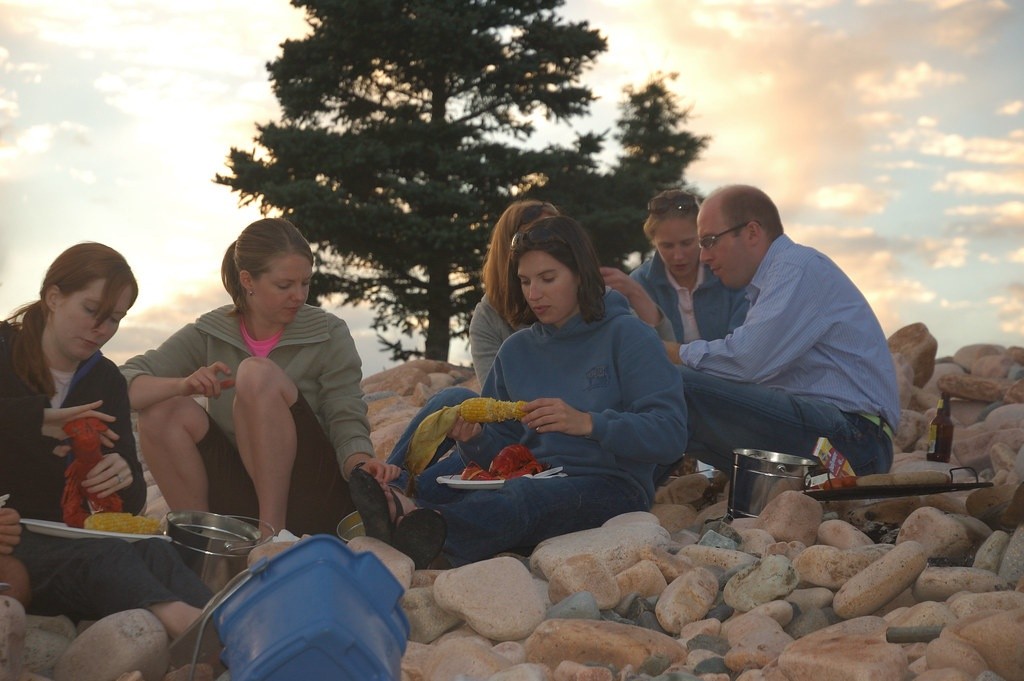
[351, 461, 365, 473]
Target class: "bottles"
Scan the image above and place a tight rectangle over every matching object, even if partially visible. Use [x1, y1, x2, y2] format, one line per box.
[926, 391, 954, 464]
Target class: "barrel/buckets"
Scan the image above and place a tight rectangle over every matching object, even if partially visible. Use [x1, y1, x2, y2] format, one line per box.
[165, 510, 276, 594]
[726, 448, 819, 519]
[337, 510, 366, 543]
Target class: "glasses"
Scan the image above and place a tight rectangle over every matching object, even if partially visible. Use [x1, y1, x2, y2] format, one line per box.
[648, 194, 697, 213]
[697, 219, 761, 249]
[512, 228, 568, 248]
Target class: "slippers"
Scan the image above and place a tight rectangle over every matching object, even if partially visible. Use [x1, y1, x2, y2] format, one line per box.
[348, 467, 449, 570]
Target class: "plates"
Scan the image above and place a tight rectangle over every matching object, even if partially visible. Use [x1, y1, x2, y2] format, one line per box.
[435, 472, 569, 490]
[20, 518, 172, 545]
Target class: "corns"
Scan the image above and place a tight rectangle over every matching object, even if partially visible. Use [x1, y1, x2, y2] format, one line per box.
[460, 398, 532, 422]
[82, 514, 164, 534]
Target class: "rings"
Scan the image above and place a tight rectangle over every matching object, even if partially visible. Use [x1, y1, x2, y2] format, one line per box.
[118, 475, 124, 483]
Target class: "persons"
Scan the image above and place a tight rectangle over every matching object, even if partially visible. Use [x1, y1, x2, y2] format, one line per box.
[0, 240, 219, 639]
[117, 217, 401, 545]
[350, 183, 900, 571]
[0, 505, 31, 602]
